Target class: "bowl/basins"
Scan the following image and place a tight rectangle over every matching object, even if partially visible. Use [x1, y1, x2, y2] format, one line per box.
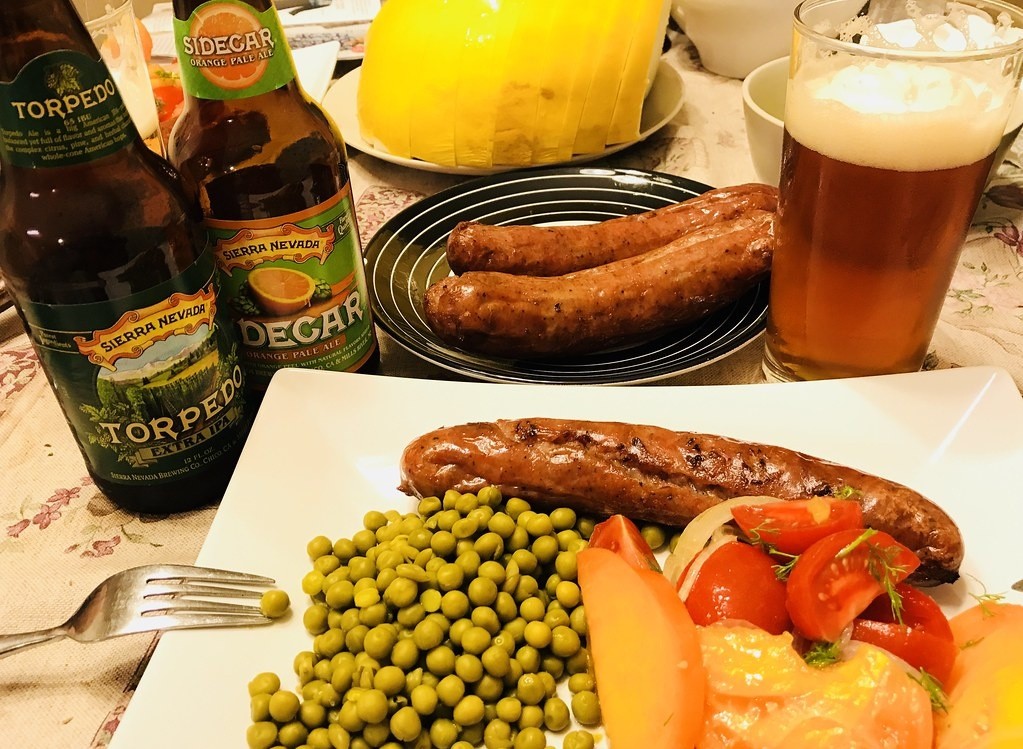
[741, 56, 1022, 189]
[672, 0, 871, 78]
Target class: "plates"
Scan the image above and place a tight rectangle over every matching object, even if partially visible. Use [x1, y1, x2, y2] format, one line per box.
[108, 366, 1023, 748]
[321, 57, 685, 176]
[276, 0, 380, 60]
[361, 166, 766, 386]
[289, 40, 341, 105]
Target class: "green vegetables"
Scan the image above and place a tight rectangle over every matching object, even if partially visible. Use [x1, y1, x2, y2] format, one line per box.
[646, 484, 1009, 715]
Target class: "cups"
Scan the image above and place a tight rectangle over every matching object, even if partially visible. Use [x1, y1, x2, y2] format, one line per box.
[73, 0, 167, 163]
[761, 0, 1023, 384]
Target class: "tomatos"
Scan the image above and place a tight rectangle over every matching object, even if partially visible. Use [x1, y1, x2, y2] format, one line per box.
[146, 61, 183, 124]
[585, 494, 955, 687]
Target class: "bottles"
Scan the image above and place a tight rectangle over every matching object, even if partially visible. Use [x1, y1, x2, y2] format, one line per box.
[0, 0, 257, 516]
[165, 0, 381, 417]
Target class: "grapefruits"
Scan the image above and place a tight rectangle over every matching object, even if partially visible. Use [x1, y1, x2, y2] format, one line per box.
[359, 0, 674, 163]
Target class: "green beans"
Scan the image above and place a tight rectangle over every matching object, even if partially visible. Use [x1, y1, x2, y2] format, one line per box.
[247, 487, 686, 749]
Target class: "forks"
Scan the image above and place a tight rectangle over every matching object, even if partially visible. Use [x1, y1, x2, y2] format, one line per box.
[0, 563, 276, 661]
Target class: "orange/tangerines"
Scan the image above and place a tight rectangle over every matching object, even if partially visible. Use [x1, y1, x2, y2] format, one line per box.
[248, 267, 315, 315]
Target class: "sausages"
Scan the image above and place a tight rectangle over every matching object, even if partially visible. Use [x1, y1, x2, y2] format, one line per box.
[419, 179, 784, 351]
[397, 417, 964, 586]
